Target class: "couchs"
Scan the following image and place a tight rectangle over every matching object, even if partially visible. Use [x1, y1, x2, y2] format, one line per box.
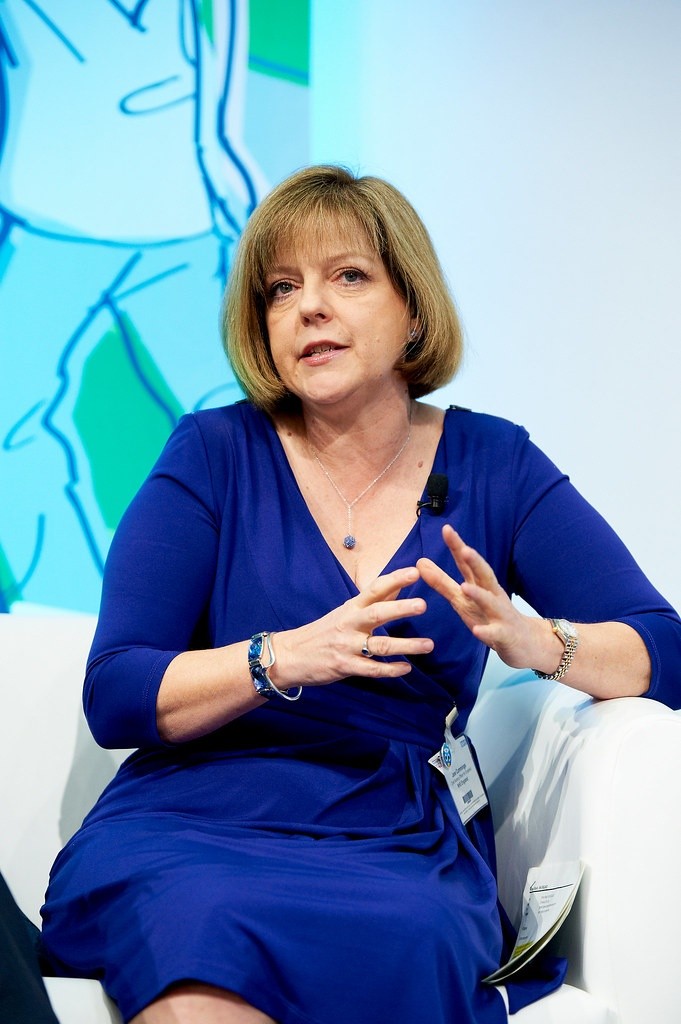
[0, 599, 681, 1024]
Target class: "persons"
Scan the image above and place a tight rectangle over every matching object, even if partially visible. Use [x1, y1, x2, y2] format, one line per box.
[29, 158, 680, 1024]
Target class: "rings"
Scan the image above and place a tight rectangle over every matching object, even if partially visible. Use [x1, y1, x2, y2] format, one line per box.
[361, 633, 376, 659]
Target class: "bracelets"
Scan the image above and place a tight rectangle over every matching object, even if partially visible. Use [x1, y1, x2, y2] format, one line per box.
[242, 625, 304, 706]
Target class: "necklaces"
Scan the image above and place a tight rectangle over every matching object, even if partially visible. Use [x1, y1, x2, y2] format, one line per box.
[289, 396, 421, 550]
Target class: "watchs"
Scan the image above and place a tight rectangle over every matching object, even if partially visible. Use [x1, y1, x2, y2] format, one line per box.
[529, 612, 579, 682]
